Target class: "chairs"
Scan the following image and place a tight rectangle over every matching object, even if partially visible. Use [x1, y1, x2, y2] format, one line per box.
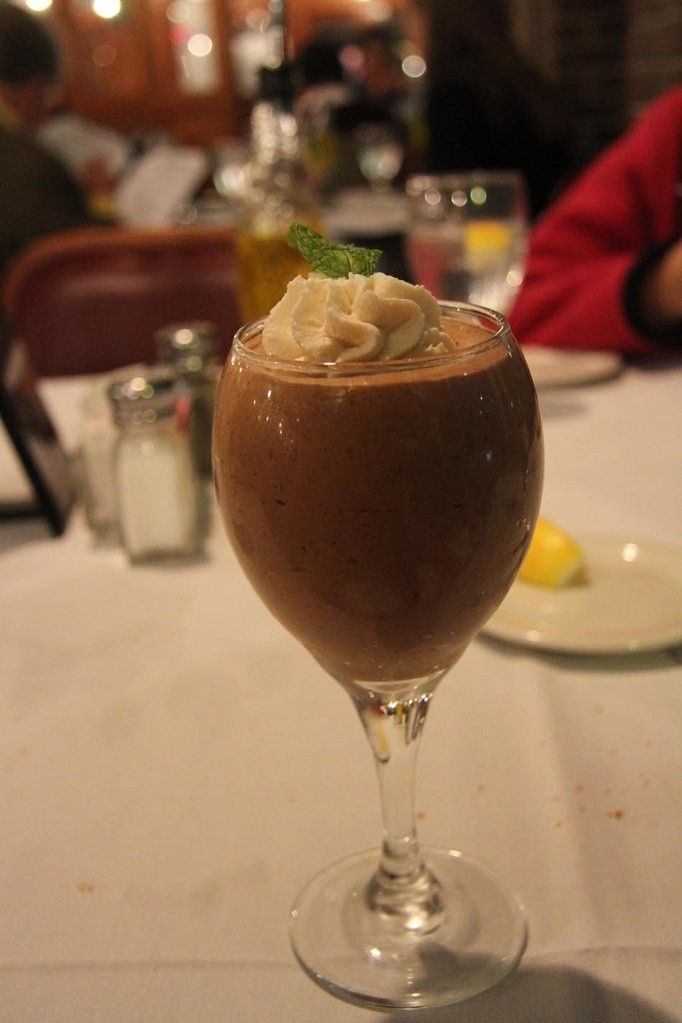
[5, 222, 293, 433]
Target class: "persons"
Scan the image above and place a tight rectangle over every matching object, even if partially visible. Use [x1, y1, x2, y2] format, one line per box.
[498, 79, 682, 368]
[0, 0, 130, 303]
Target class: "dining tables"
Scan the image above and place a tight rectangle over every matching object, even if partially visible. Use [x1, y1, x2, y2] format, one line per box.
[0, 183, 420, 239]
[2, 339, 680, 1020]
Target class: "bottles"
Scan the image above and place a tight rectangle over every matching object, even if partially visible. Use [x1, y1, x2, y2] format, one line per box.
[109, 369, 204, 560]
[158, 320, 224, 476]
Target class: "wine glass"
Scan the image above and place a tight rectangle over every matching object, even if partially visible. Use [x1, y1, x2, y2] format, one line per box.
[407, 174, 526, 321]
[210, 296, 529, 1008]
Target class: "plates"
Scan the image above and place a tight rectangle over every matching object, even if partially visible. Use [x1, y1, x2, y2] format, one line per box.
[507, 345, 624, 384]
[476, 519, 682, 658]
